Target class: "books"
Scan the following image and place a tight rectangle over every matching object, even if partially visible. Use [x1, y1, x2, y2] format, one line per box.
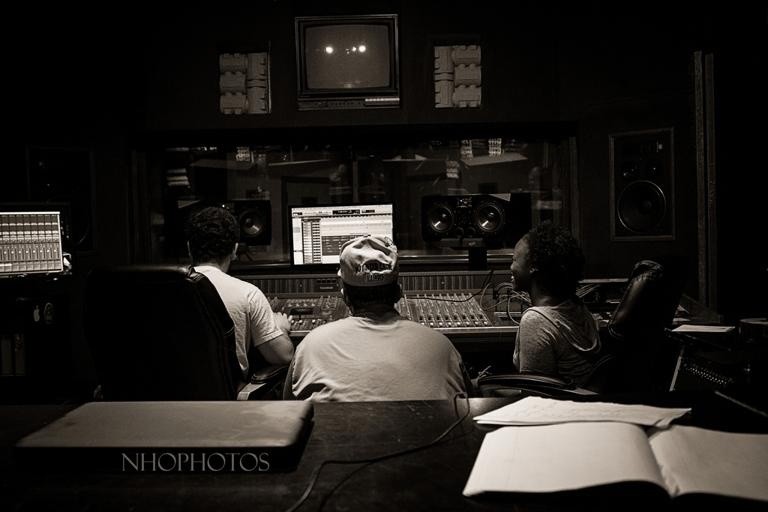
[462, 420, 768, 502]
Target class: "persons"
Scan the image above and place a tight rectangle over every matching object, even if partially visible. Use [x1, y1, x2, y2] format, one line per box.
[181, 206, 293, 401]
[467, 217, 608, 396]
[282, 231, 475, 403]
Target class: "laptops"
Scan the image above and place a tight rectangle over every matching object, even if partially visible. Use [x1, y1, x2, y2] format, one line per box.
[13, 398, 316, 472]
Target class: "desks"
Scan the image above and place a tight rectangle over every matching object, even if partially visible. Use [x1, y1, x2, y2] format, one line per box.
[1, 403, 767, 510]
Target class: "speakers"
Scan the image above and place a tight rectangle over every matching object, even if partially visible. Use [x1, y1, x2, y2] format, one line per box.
[606, 126, 679, 243]
[420, 190, 532, 250]
[231, 197, 273, 247]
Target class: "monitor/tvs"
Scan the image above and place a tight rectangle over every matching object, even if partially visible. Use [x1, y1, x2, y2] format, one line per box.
[293, 12, 401, 111]
[289, 201, 398, 269]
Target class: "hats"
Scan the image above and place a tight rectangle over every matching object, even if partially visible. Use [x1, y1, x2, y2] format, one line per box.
[336, 234, 398, 287]
